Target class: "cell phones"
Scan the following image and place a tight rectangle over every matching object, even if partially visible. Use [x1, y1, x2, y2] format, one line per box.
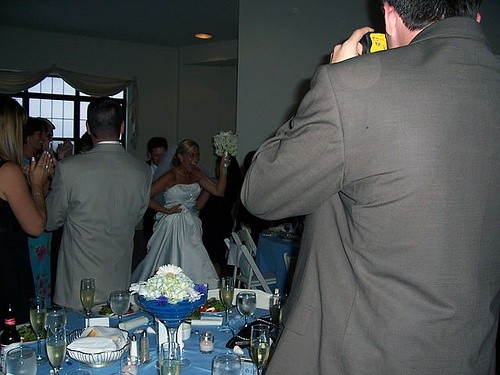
[365, 32, 387, 54]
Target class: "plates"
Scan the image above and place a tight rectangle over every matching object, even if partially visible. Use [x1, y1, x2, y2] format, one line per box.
[0, 322, 50, 347]
[200, 305, 236, 320]
[91, 302, 139, 318]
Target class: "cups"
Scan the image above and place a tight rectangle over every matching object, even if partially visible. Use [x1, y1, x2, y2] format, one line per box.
[182, 323, 191, 340]
[120, 355, 138, 375]
[5, 347, 37, 375]
[160, 342, 181, 375]
[213, 353, 243, 375]
[269, 295, 282, 326]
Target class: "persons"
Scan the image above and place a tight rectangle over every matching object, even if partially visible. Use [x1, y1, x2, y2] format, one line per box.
[44, 98, 154, 312]
[130, 139, 230, 290]
[132, 137, 304, 298]
[21, 116, 96, 309]
[240, 0, 500, 375]
[0, 93, 53, 327]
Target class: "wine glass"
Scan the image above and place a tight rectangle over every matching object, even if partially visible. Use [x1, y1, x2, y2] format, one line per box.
[46, 305, 67, 341]
[237, 291, 256, 328]
[30, 296, 49, 366]
[251, 325, 270, 375]
[46, 324, 67, 375]
[220, 277, 234, 332]
[110, 292, 130, 324]
[80, 278, 95, 318]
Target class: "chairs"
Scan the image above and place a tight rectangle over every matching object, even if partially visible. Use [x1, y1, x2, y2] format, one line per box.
[223, 223, 298, 294]
[208, 288, 281, 310]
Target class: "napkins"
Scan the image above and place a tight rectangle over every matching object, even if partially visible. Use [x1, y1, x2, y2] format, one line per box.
[191, 317, 223, 325]
[119, 317, 149, 330]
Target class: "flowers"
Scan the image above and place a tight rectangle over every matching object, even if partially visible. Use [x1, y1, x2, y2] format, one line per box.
[214, 130, 239, 176]
[129, 263, 205, 304]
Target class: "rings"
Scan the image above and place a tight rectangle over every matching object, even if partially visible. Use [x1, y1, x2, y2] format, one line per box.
[45, 165, 49, 169]
[229, 160, 230, 162]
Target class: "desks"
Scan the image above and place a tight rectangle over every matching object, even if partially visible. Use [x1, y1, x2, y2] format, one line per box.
[22, 304, 269, 375]
[255, 235, 300, 297]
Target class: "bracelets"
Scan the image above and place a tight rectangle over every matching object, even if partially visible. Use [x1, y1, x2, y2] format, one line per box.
[34, 193, 41, 196]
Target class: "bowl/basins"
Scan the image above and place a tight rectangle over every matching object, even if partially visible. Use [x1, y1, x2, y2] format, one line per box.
[66, 329, 130, 368]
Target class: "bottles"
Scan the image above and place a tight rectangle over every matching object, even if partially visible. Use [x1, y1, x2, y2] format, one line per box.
[0, 303, 22, 375]
[130, 335, 139, 365]
[141, 331, 150, 363]
[199, 332, 214, 354]
